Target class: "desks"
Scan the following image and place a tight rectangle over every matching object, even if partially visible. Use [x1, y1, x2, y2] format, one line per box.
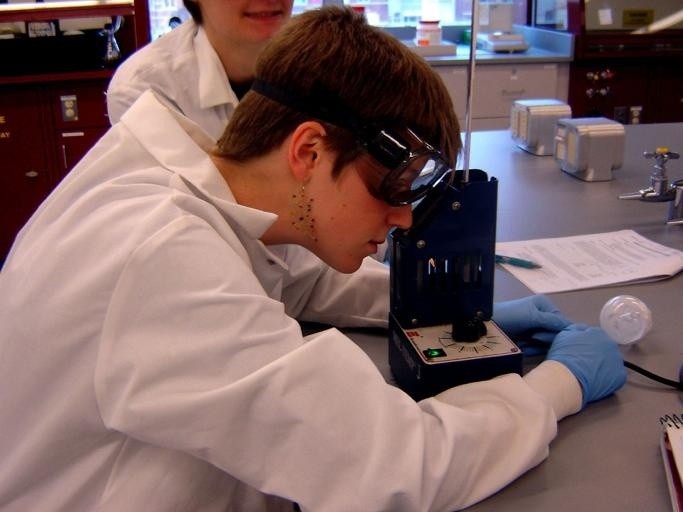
[265, 121, 682, 512]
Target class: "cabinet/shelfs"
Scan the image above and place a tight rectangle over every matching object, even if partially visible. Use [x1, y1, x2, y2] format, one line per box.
[457, 65, 560, 133]
[0, 1, 154, 269]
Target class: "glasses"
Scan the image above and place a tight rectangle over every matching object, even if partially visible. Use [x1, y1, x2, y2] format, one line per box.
[351, 115, 452, 207]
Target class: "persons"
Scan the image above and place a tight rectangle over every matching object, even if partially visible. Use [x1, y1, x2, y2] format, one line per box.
[105, 0, 294, 145]
[0, 7, 627, 511]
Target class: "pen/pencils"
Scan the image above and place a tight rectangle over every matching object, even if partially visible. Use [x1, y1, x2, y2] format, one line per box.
[494, 256, 544, 269]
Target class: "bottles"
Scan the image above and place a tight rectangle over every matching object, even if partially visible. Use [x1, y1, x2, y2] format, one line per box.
[416, 20, 441, 45]
